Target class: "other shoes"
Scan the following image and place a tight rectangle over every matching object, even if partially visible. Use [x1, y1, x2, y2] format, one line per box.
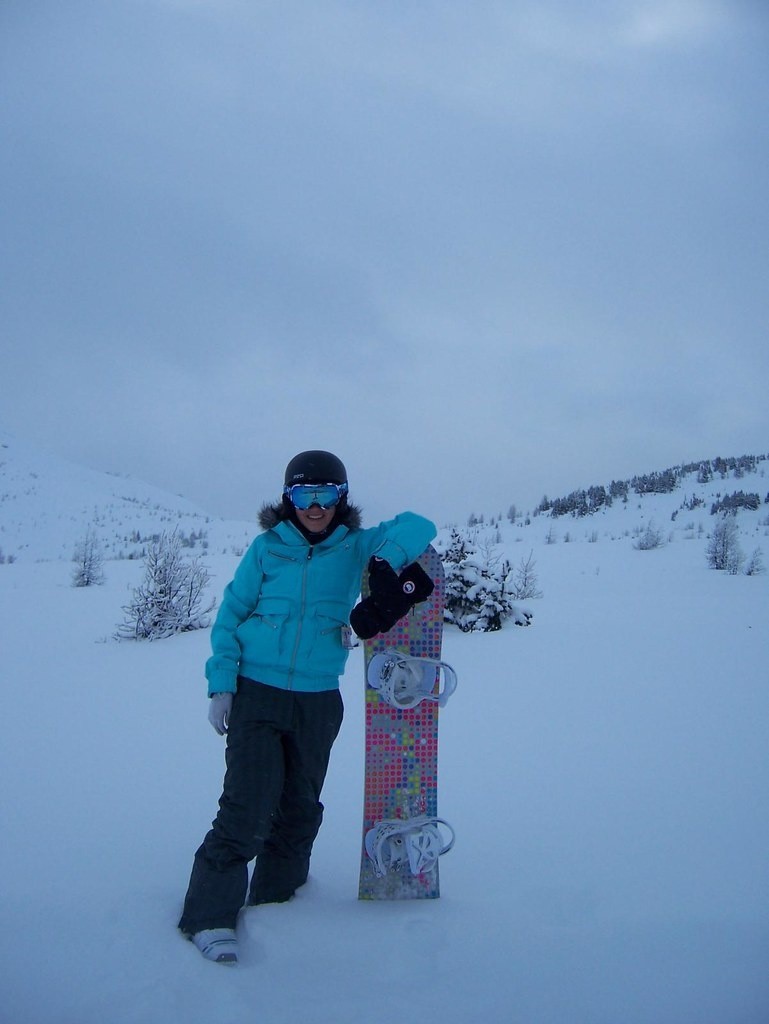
[189, 927, 240, 965]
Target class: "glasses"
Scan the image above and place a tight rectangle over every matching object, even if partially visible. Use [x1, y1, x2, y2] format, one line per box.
[290, 483, 341, 512]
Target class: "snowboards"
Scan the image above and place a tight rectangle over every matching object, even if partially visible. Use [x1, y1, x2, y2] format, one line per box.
[358, 541, 459, 901]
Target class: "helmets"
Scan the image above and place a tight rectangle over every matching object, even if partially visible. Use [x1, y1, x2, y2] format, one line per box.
[285, 450, 347, 491]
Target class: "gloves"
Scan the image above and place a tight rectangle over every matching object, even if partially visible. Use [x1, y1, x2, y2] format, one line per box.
[349, 556, 437, 641]
[207, 692, 235, 736]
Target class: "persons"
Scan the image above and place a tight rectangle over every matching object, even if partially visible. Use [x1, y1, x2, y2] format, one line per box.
[175, 449, 438, 962]
